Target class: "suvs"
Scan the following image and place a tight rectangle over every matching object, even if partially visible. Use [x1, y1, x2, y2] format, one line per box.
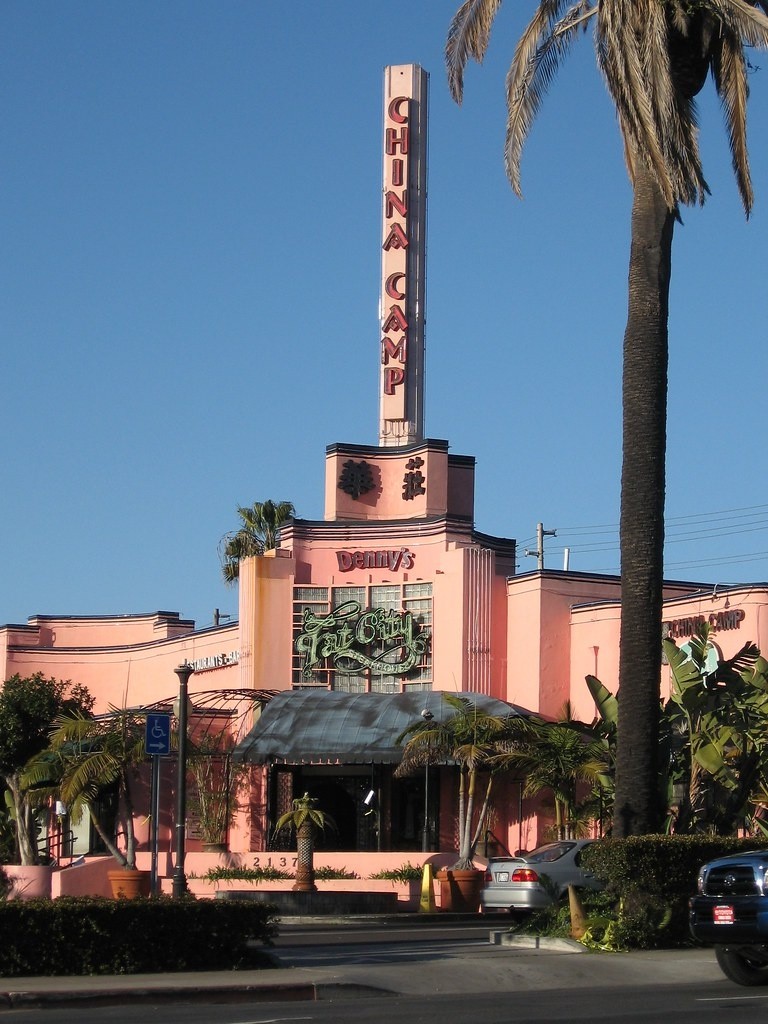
[688, 842, 768, 990]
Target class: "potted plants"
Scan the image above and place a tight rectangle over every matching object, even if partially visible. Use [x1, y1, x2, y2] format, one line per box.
[0, 671, 95, 901]
[174, 729, 249, 852]
[393, 691, 507, 913]
[14, 692, 204, 899]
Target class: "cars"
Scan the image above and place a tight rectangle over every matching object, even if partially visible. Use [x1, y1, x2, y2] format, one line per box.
[479, 836, 613, 924]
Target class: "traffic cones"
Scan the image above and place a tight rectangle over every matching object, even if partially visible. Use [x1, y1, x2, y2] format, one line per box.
[567, 882, 590, 943]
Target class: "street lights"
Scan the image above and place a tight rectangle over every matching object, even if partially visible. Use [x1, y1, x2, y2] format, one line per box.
[172, 658, 195, 899]
[420, 708, 434, 852]
